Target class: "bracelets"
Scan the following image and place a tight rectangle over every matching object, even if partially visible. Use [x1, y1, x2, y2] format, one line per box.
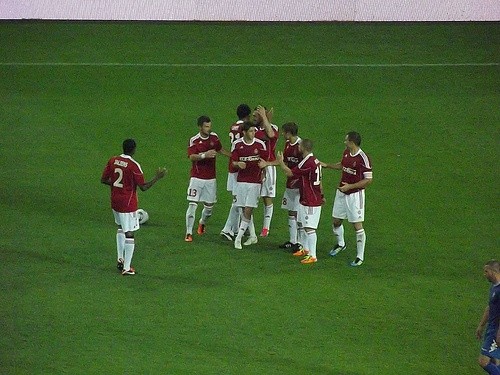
[199, 152, 205, 160]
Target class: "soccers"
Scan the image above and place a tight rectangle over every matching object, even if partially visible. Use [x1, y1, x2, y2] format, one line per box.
[136, 208, 150, 225]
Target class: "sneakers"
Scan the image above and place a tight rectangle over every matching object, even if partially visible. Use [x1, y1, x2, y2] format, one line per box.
[329, 242, 346, 256]
[293, 249, 309, 256]
[234, 237, 242, 249]
[198, 223, 206, 234]
[117, 262, 124, 269]
[220, 231, 235, 241]
[279, 241, 294, 249]
[122, 268, 135, 274]
[301, 256, 317, 264]
[243, 236, 258, 245]
[292, 243, 303, 253]
[184, 235, 192, 241]
[351, 257, 364, 266]
[260, 228, 270, 237]
[234, 232, 239, 236]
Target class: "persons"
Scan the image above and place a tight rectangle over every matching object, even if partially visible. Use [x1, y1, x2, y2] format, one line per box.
[185, 114, 232, 242]
[219, 104, 373, 267]
[475, 259, 500, 375]
[100, 138, 168, 275]
